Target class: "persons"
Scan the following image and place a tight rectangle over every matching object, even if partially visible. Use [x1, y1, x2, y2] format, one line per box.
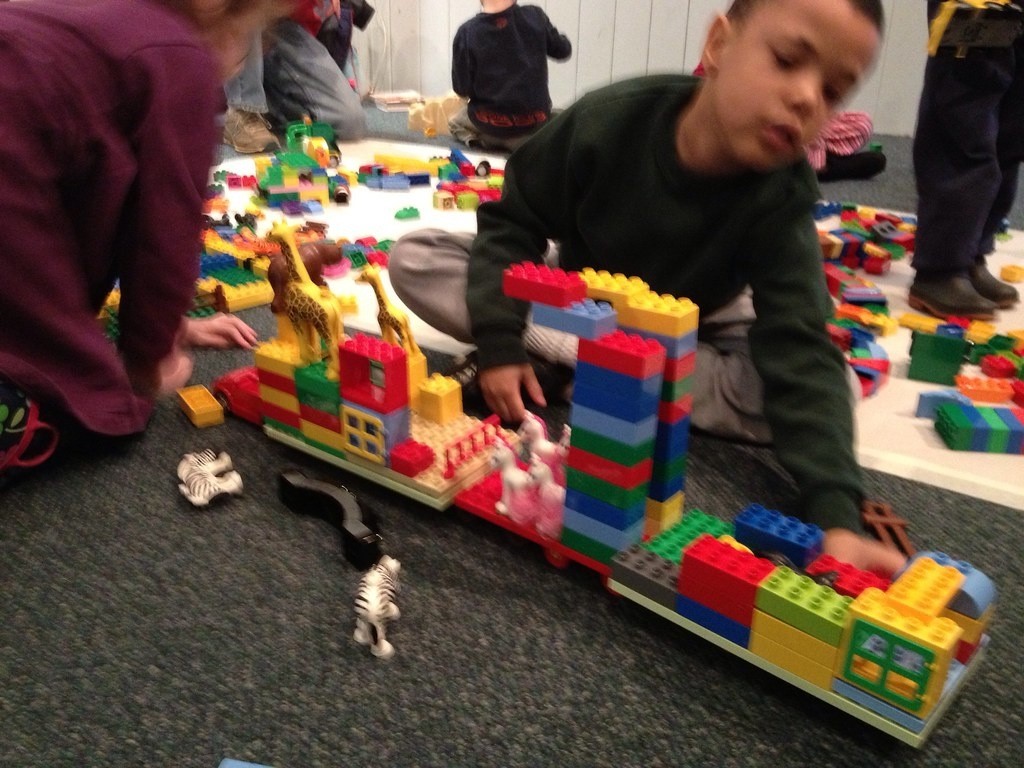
[446, 0, 571, 155]
[0, 0, 374, 436]
[387, 0, 907, 581]
[798, 103, 885, 185]
[909, 0, 1024, 323]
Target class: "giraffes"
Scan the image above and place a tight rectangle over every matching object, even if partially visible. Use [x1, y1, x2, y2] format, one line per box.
[355, 265, 420, 357]
[265, 218, 349, 382]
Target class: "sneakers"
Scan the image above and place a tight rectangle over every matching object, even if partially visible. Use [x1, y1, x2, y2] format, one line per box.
[222, 107, 281, 153]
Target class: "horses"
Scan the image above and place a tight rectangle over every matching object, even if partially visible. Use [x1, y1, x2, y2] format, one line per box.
[487, 411, 572, 540]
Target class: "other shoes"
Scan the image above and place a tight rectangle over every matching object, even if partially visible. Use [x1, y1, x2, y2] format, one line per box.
[909, 277, 994, 320]
[441, 350, 570, 416]
[970, 264, 1018, 306]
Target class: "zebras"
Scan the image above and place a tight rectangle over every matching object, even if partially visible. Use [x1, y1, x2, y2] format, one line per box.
[354, 554, 403, 659]
[177, 449, 244, 506]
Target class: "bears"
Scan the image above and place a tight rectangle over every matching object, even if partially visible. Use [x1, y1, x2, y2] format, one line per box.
[268, 244, 344, 316]
